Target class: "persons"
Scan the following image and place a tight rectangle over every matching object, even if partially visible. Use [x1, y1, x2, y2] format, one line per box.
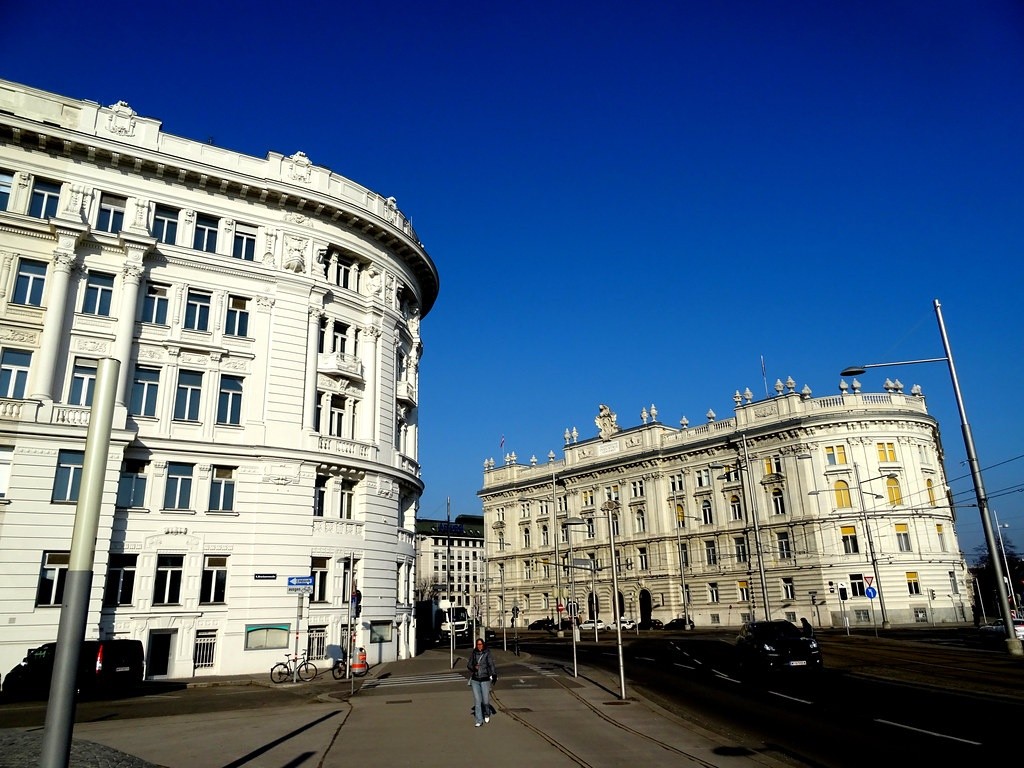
[467, 638, 497, 726]
[801, 618, 812, 638]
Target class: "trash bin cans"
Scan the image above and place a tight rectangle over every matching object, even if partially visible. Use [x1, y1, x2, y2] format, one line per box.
[352, 647, 366, 673]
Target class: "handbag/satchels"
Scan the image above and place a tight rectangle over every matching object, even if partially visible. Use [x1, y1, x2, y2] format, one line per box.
[467, 677, 472, 686]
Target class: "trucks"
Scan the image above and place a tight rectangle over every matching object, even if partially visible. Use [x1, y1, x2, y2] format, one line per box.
[442, 606, 469, 644]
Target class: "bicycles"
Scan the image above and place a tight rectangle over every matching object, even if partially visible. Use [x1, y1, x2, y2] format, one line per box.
[332, 653, 369, 681]
[270, 648, 318, 683]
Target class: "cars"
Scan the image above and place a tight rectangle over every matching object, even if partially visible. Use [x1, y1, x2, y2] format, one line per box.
[528, 620, 553, 630]
[469, 618, 497, 643]
[638, 619, 663, 631]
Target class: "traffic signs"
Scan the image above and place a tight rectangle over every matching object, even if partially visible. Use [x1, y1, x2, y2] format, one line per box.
[288, 577, 313, 585]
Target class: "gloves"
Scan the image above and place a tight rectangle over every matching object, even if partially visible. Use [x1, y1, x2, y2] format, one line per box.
[491, 674, 497, 686]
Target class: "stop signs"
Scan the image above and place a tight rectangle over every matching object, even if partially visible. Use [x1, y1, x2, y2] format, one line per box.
[558, 604, 563, 611]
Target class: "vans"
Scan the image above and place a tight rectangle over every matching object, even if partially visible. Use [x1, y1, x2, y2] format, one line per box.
[1, 639, 145, 701]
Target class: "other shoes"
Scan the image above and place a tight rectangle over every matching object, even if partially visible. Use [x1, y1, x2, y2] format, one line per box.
[485, 716, 490, 723]
[475, 722, 483, 727]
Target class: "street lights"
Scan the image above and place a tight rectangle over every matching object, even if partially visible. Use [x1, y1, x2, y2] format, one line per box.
[562, 518, 586, 678]
[809, 473, 903, 630]
[518, 496, 565, 637]
[676, 512, 705, 629]
[997, 523, 1022, 619]
[337, 556, 354, 679]
[844, 353, 1024, 656]
[709, 453, 813, 621]
[485, 540, 511, 628]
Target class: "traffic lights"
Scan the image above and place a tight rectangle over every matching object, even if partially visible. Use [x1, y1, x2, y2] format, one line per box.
[930, 589, 936, 600]
[530, 558, 537, 573]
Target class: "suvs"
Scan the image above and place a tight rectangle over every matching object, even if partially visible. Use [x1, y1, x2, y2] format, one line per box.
[605, 616, 637, 630]
[979, 619, 1024, 641]
[737, 620, 824, 678]
[554, 617, 579, 630]
[664, 618, 694, 630]
[579, 619, 605, 631]
